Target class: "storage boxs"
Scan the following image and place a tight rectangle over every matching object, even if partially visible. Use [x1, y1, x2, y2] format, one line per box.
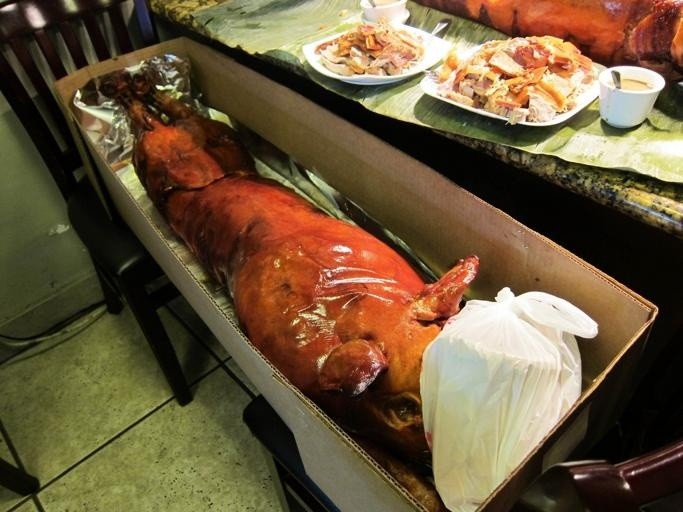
[52, 39, 661, 512]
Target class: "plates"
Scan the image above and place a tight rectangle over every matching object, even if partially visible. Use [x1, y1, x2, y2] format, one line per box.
[419, 43, 603, 127]
[302, 22, 453, 87]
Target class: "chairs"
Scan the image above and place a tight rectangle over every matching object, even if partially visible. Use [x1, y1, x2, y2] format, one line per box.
[0, 0, 193, 405]
[243, 391, 341, 512]
[541, 442, 683, 510]
[1, 458, 39, 498]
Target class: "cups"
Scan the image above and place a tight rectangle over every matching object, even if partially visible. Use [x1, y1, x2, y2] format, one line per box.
[598, 65, 666, 128]
[360, 0, 407, 22]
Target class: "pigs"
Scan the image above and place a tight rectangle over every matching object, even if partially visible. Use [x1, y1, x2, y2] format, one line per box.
[96, 66, 482, 472]
